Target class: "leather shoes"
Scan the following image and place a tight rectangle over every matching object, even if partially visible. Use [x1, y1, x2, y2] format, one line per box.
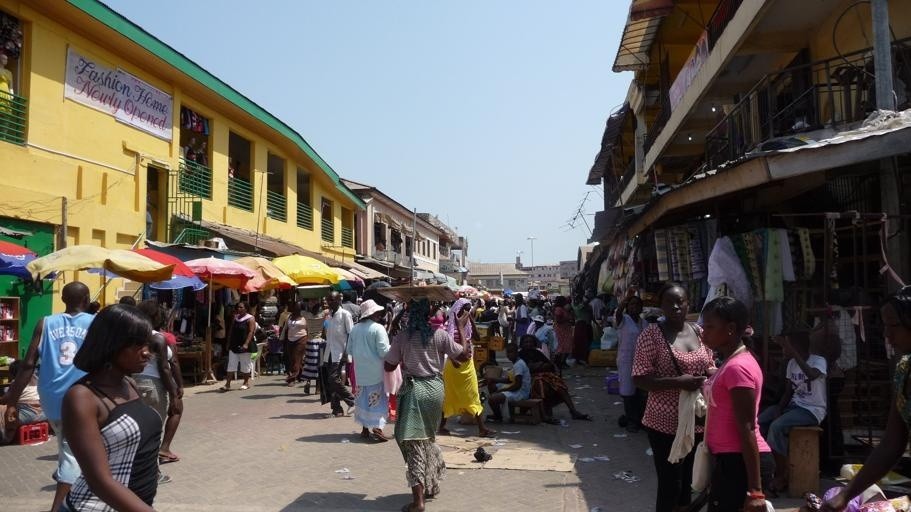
[572, 414, 588, 420]
[543, 415, 561, 423]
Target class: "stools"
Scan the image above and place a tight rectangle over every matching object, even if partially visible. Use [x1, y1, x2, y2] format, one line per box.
[17, 421, 50, 445]
[270, 352, 284, 375]
[606, 373, 620, 396]
[784, 424, 824, 499]
[509, 397, 545, 425]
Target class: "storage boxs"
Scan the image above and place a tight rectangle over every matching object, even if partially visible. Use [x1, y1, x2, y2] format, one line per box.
[485, 364, 504, 379]
[476, 325, 491, 339]
[474, 346, 496, 361]
[490, 337, 505, 352]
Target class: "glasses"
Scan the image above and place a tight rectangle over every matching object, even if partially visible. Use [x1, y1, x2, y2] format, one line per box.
[897, 285, 911, 302]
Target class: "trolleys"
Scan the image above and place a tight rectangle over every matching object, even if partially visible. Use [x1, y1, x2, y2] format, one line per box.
[467, 319, 502, 377]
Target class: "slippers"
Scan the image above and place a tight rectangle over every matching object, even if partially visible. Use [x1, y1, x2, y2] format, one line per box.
[220, 386, 229, 392]
[239, 385, 250, 390]
[401, 503, 422, 512]
[157, 474, 173, 484]
[425, 488, 435, 499]
[360, 431, 371, 441]
[373, 432, 388, 442]
[158, 453, 179, 464]
[479, 430, 497, 437]
[439, 429, 449, 435]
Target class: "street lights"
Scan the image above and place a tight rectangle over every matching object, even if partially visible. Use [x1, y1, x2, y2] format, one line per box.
[526, 235, 537, 273]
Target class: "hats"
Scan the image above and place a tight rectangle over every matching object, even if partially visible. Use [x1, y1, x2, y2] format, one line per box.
[530, 295, 542, 301]
[358, 298, 385, 321]
[533, 315, 544, 322]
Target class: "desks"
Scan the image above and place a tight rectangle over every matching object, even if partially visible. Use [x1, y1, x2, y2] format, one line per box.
[175, 349, 215, 385]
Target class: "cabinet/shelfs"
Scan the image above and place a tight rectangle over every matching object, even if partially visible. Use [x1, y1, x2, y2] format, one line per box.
[0, 296, 21, 396]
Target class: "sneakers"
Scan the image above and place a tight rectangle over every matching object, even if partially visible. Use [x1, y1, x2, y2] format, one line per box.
[323, 412, 336, 419]
[347, 404, 356, 417]
[288, 382, 295, 387]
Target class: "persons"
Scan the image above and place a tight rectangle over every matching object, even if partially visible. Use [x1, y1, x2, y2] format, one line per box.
[184, 137, 197, 171]
[197, 142, 208, 166]
[228, 169, 235, 177]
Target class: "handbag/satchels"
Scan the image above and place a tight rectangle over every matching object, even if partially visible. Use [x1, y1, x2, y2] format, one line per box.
[690, 442, 711, 493]
[694, 414, 711, 428]
[267, 337, 281, 353]
